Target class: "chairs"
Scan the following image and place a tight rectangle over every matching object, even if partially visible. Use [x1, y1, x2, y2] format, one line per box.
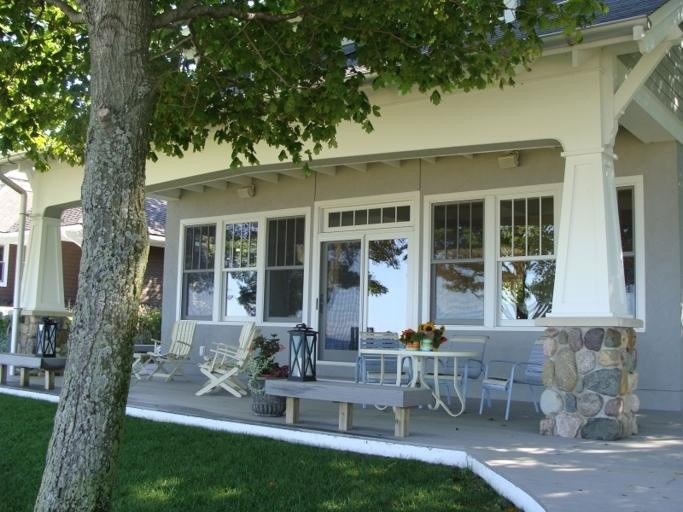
[355, 331, 406, 409]
[131, 353, 150, 380]
[195, 322, 256, 398]
[426, 336, 492, 412]
[479, 336, 549, 420]
[147, 318, 197, 382]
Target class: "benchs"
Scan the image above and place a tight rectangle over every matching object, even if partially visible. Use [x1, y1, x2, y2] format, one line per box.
[0, 352, 66, 391]
[265, 378, 431, 438]
[134, 344, 159, 364]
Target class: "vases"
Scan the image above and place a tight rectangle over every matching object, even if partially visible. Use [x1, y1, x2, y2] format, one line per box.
[250, 376, 286, 417]
[405, 339, 439, 352]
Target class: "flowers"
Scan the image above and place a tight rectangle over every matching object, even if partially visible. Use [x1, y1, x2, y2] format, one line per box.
[249, 333, 288, 394]
[400, 322, 447, 348]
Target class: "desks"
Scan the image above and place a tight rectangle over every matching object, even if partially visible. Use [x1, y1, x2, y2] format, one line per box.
[359, 348, 476, 417]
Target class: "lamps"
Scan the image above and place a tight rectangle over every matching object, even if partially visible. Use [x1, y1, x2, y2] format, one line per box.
[497, 150, 519, 169]
[288, 323, 320, 382]
[238, 184, 255, 199]
[35, 317, 56, 357]
